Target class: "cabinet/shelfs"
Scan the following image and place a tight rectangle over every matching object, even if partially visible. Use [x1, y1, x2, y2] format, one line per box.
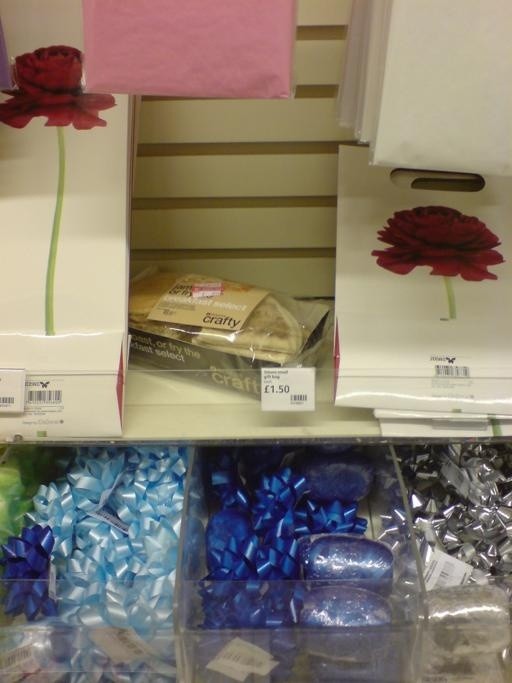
[0, 2, 512, 682]
